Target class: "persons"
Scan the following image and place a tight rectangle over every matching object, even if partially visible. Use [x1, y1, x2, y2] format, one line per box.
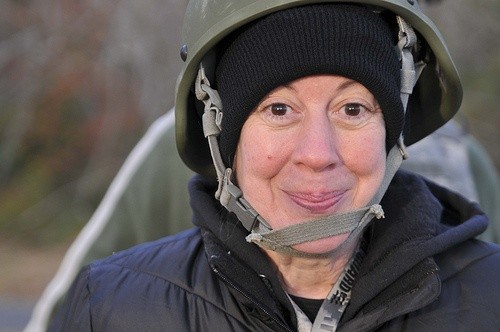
[53, 0, 500, 332]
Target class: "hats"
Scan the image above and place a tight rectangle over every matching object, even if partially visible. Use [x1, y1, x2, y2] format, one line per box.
[210, 5, 404, 175]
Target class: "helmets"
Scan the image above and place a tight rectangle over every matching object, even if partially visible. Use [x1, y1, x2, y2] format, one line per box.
[174, 2, 463, 175]
